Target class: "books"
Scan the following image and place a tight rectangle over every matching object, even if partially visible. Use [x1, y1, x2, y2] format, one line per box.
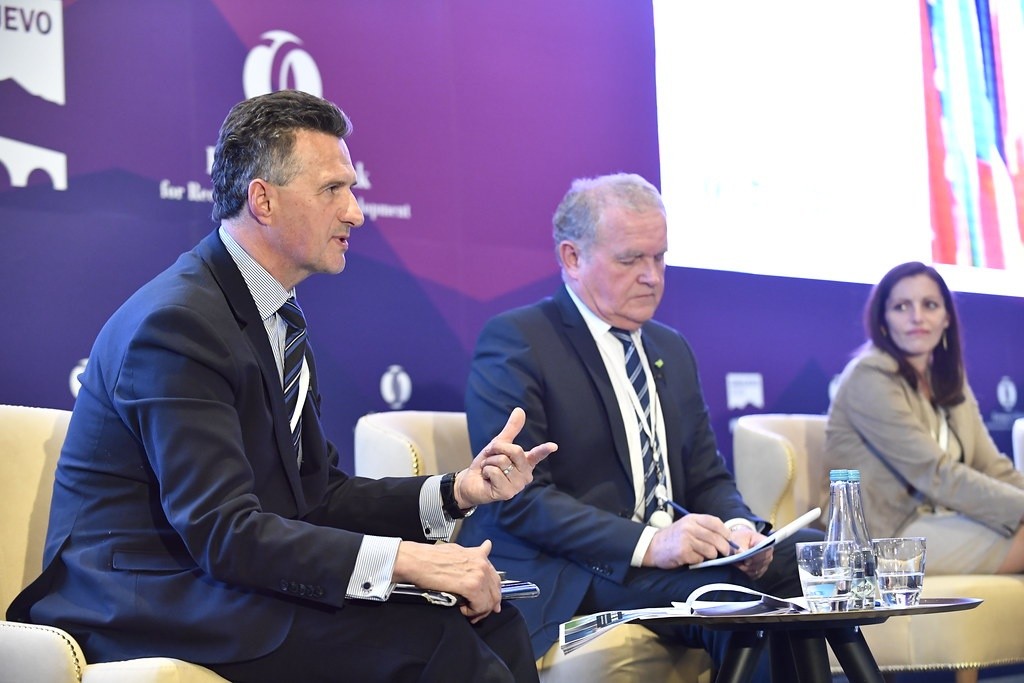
[390, 577, 542, 606]
[559, 583, 809, 656]
[689, 507, 821, 569]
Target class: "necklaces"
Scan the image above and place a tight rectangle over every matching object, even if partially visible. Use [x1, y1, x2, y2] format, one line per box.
[594, 329, 673, 528]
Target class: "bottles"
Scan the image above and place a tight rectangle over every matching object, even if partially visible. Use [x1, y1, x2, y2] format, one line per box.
[823, 468, 865, 610]
[846, 468, 875, 609]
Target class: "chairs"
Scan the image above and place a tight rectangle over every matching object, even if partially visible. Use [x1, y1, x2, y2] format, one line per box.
[353, 409, 713, 683]
[736, 412, 1024, 683]
[0, 404, 229, 683]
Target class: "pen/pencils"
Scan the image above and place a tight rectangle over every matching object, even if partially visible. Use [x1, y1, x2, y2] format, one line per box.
[667, 501, 745, 552]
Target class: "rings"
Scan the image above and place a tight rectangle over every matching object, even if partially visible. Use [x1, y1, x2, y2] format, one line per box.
[503, 463, 515, 474]
[755, 570, 758, 574]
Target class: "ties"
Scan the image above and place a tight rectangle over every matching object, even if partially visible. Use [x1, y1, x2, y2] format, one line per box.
[278, 298, 311, 470]
[610, 326, 668, 523]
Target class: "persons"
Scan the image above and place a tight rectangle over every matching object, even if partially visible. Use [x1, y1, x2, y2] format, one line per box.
[7, 90, 541, 683]
[819, 262, 1024, 577]
[456, 172, 878, 683]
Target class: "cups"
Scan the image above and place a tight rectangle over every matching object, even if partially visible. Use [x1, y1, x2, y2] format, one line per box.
[795, 541, 854, 614]
[871, 537, 927, 608]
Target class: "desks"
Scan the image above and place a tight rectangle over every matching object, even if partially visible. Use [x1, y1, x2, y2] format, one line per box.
[628, 598, 985, 683]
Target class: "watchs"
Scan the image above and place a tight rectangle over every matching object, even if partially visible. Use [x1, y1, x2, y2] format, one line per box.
[440, 471, 478, 519]
